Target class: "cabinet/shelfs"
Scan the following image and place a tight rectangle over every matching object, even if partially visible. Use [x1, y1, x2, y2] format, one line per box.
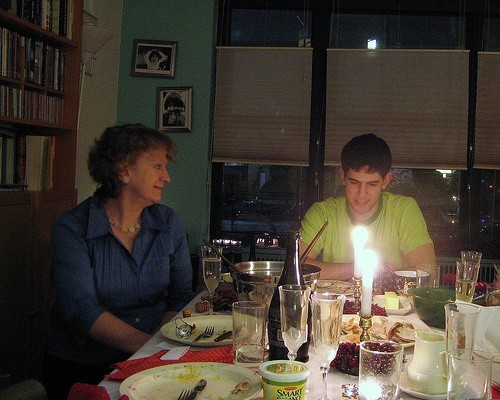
[0, 0, 86, 390]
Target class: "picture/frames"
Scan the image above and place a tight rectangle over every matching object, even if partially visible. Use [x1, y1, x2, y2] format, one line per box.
[155, 86, 193, 132]
[131, 38, 179, 78]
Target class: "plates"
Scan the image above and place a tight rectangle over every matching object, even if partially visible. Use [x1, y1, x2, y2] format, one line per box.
[341, 315, 437, 348]
[160, 314, 262, 347]
[316, 279, 355, 296]
[373, 293, 412, 315]
[119, 361, 263, 400]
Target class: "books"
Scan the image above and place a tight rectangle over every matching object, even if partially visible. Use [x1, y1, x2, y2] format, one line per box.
[0, 27, 64, 92]
[0, 123, 59, 191]
[0, 84, 63, 125]
[0, 0, 73, 40]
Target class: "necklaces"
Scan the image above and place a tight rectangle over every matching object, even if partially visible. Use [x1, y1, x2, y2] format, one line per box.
[104, 199, 141, 233]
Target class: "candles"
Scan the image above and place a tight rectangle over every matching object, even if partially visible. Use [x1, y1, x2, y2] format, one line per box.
[352, 227, 372, 280]
[362, 259, 377, 319]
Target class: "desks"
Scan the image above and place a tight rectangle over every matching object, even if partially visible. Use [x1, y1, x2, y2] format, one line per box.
[93, 270, 500, 400]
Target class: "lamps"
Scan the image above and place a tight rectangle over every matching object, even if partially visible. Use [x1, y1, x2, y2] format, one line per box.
[77, 24, 115, 125]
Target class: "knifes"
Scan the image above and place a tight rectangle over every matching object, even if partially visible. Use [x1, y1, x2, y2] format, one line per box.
[186, 379, 207, 400]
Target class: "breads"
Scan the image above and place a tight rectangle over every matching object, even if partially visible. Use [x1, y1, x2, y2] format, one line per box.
[341, 315, 417, 344]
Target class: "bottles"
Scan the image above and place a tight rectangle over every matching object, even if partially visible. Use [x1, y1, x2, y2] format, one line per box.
[267, 231, 311, 363]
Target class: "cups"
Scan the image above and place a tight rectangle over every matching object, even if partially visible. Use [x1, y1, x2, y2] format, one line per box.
[258, 360, 310, 400]
[454, 250, 483, 303]
[446, 348, 494, 400]
[415, 263, 440, 289]
[405, 330, 454, 394]
[443, 301, 482, 369]
[358, 340, 404, 400]
[231, 299, 268, 369]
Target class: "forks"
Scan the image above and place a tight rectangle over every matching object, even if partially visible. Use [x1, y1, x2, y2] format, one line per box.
[192, 326, 214, 341]
[177, 389, 193, 400]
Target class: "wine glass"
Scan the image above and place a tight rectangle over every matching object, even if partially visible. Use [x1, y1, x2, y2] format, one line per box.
[278, 284, 311, 360]
[310, 292, 343, 400]
[201, 244, 222, 315]
[398, 370, 456, 400]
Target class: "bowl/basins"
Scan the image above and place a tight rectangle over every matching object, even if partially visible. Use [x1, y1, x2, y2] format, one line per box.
[392, 270, 431, 290]
[228, 261, 322, 317]
[406, 287, 456, 329]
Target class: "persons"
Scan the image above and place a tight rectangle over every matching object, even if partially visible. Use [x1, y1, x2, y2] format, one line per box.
[260, 166, 294, 204]
[163, 92, 185, 126]
[299, 135, 437, 283]
[50, 122, 194, 400]
[143, 49, 168, 70]
